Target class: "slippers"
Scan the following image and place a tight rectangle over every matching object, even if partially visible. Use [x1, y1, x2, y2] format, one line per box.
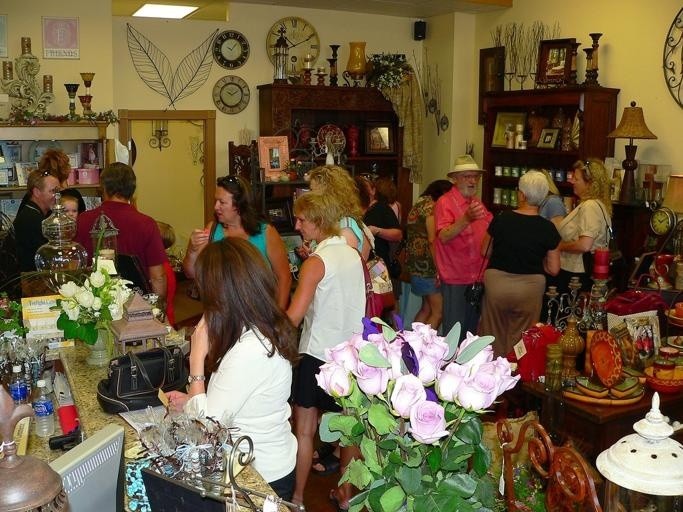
[312, 442, 335, 462]
[312, 453, 341, 476]
[328, 488, 351, 512]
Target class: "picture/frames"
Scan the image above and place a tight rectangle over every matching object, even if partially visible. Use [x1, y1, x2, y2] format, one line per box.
[538, 128, 558, 149]
[490, 109, 527, 150]
[67, 154, 78, 170]
[365, 122, 394, 154]
[0, 169, 8, 185]
[478, 47, 505, 125]
[605, 158, 639, 197]
[17, 162, 39, 186]
[538, 43, 572, 82]
[67, 168, 75, 186]
[76, 168, 98, 184]
[3, 145, 21, 164]
[258, 136, 289, 179]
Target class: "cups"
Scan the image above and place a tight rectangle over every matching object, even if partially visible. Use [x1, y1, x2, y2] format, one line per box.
[653, 359, 675, 380]
[658, 347, 679, 364]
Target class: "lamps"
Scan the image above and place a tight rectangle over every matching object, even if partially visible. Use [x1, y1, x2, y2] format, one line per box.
[661, 175, 683, 213]
[606, 101, 658, 203]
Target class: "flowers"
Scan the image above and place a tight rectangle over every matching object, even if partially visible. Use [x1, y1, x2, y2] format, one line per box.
[317, 316, 523, 512]
[48, 269, 133, 344]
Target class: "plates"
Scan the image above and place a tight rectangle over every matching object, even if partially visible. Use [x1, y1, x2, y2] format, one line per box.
[575, 376, 610, 398]
[317, 124, 347, 156]
[610, 376, 640, 399]
[590, 330, 624, 387]
[561, 386, 644, 406]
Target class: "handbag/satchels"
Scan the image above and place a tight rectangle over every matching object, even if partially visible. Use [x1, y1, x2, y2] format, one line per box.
[463, 210, 504, 306]
[96, 344, 192, 414]
[353, 246, 395, 334]
[352, 216, 392, 294]
[582, 201, 624, 284]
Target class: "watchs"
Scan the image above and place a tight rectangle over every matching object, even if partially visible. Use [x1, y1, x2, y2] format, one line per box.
[186, 373, 208, 385]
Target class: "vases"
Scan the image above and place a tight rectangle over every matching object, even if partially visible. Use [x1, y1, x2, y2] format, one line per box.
[84, 325, 111, 365]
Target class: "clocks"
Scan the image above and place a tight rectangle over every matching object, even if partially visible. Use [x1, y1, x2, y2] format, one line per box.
[213, 76, 249, 114]
[214, 29, 251, 69]
[266, 18, 320, 75]
[650, 207, 674, 237]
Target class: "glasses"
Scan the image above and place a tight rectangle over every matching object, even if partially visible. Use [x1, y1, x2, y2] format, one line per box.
[218, 175, 239, 189]
[36, 168, 55, 177]
[582, 158, 591, 170]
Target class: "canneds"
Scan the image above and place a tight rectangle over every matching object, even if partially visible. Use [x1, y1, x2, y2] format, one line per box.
[566, 169, 577, 183]
[547, 170, 554, 181]
[494, 166, 502, 177]
[502, 189, 510, 206]
[493, 188, 502, 204]
[512, 168, 520, 177]
[563, 196, 574, 213]
[504, 166, 511, 176]
[555, 170, 566, 181]
[521, 168, 529, 176]
[510, 191, 518, 207]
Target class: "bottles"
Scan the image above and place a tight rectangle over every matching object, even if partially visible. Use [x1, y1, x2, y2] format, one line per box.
[10, 366, 28, 408]
[515, 124, 523, 148]
[505, 128, 514, 149]
[32, 380, 54, 437]
[546, 344, 562, 393]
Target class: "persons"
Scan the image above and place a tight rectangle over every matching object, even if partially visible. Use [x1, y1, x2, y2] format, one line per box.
[155, 220, 177, 327]
[476, 168, 563, 361]
[295, 163, 376, 263]
[539, 166, 567, 229]
[403, 179, 453, 334]
[17, 148, 72, 221]
[353, 176, 405, 269]
[163, 236, 299, 504]
[433, 154, 495, 349]
[65, 161, 167, 316]
[12, 168, 61, 272]
[556, 157, 613, 320]
[284, 190, 367, 512]
[183, 173, 292, 312]
[57, 186, 87, 220]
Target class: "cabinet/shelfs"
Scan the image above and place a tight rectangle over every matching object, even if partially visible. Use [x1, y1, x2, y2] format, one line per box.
[257, 84, 414, 230]
[612, 200, 660, 294]
[0, 118, 120, 227]
[481, 88, 621, 215]
[251, 167, 354, 288]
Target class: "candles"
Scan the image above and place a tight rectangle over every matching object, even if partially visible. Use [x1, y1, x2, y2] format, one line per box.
[591, 244, 611, 278]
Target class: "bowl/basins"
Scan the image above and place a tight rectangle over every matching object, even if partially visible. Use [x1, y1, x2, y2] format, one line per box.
[645, 366, 682, 394]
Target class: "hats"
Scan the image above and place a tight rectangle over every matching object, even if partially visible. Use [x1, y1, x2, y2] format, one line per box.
[447, 154, 487, 178]
